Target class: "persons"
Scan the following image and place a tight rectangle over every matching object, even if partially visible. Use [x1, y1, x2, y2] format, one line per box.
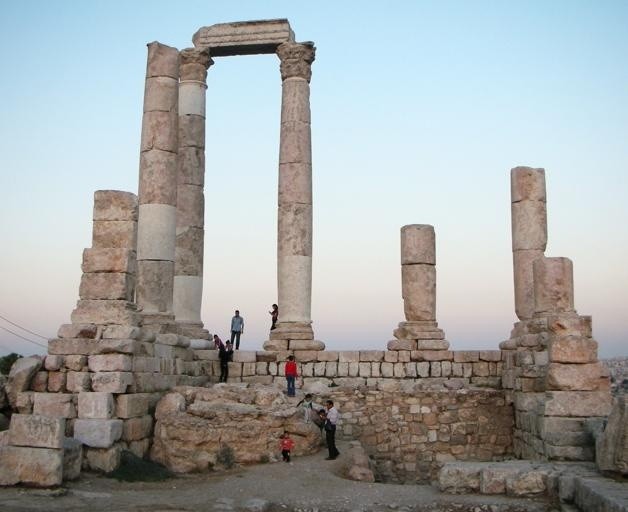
[214, 335, 233, 383]
[285, 355, 298, 397]
[295, 394, 312, 423]
[231, 310, 244, 350]
[269, 304, 278, 330]
[319, 400, 339, 460]
[279, 431, 295, 462]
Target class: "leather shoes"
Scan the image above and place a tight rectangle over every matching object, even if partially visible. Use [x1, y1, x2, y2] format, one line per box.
[325, 452, 340, 460]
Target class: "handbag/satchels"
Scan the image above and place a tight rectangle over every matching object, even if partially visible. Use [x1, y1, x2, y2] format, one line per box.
[325, 419, 333, 431]
